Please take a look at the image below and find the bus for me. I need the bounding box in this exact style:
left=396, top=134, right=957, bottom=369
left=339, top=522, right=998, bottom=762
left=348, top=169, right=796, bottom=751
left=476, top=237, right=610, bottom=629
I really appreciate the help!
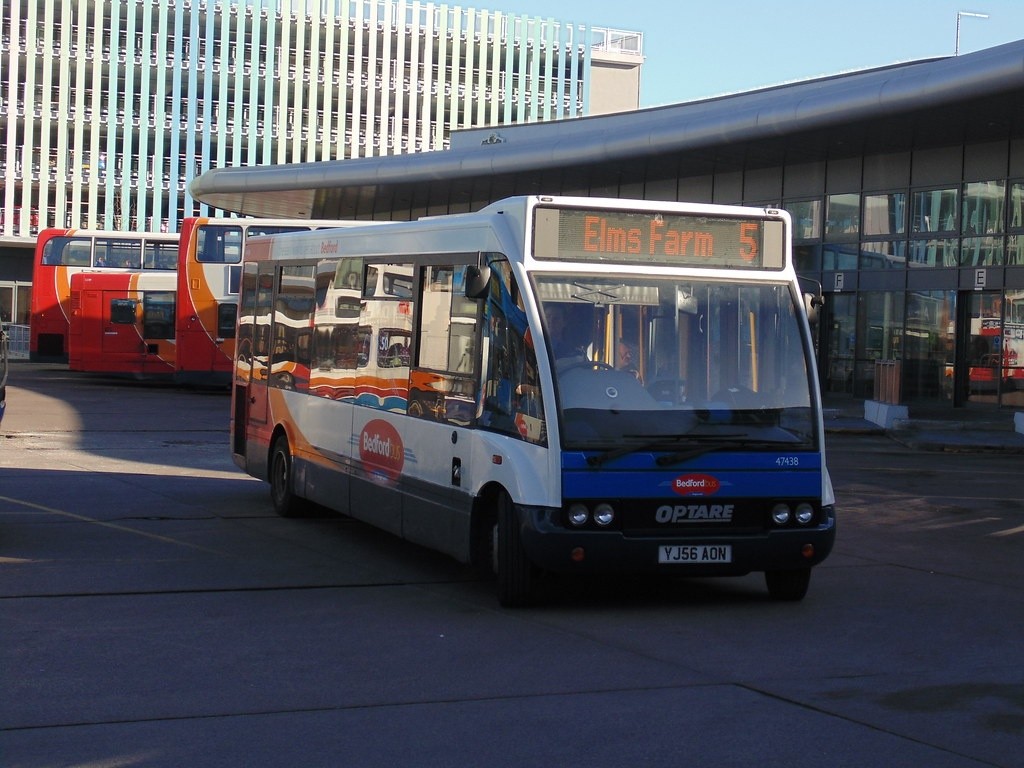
left=240, top=261, right=493, bottom=424
left=225, top=194, right=837, bottom=609
left=29, top=217, right=407, bottom=396
left=943, top=317, right=1023, bottom=392
